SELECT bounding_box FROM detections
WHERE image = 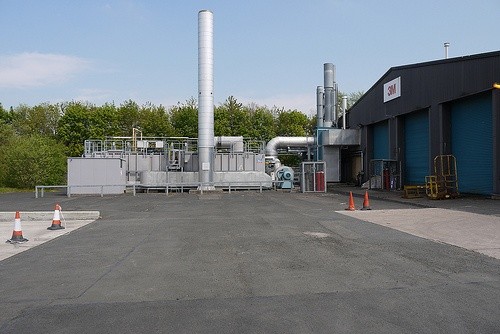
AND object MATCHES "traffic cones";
[6,210,28,244]
[360,191,372,210]
[345,192,358,211]
[47,204,65,231]
